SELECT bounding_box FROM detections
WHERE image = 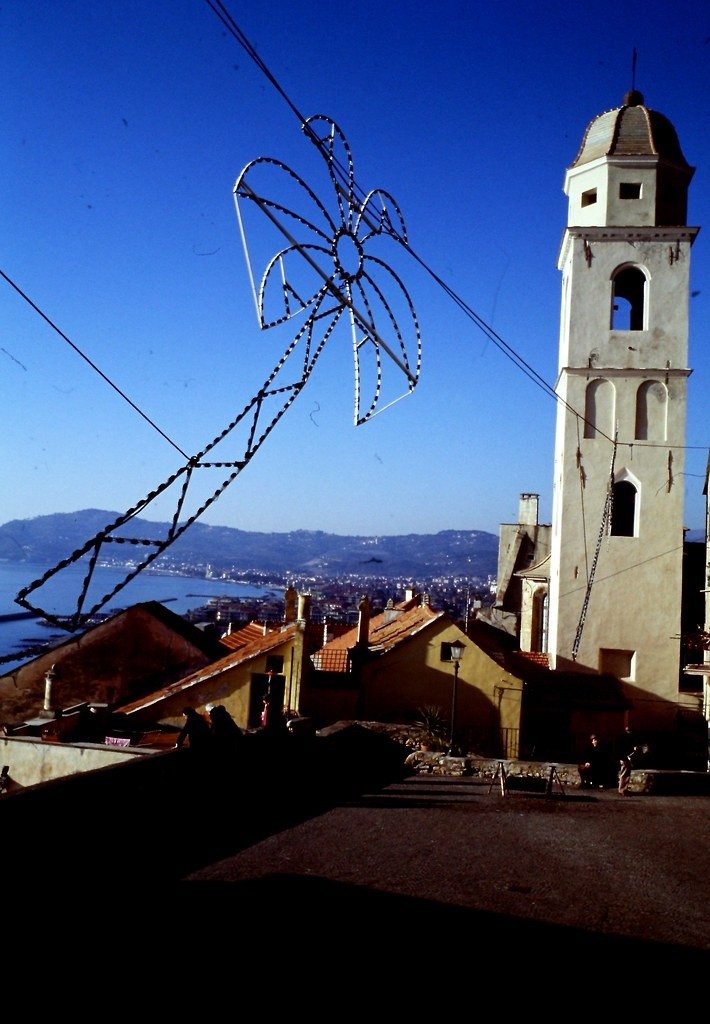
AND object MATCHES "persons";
[259,691,289,765]
[174,705,216,782]
[209,706,244,781]
[577,732,613,789]
[609,724,649,797]
